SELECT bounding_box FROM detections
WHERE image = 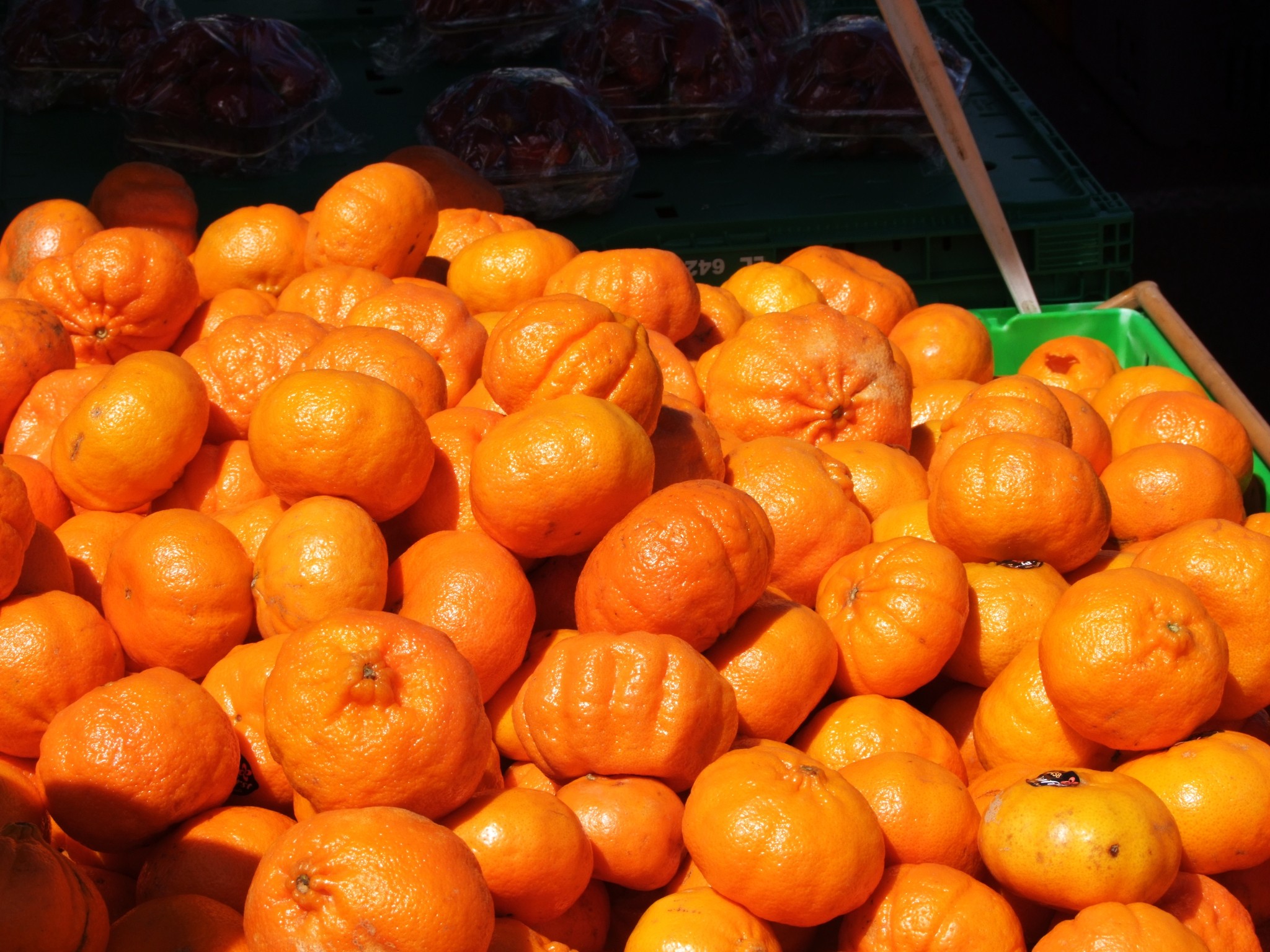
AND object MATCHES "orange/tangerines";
[0,147,1270,952]
